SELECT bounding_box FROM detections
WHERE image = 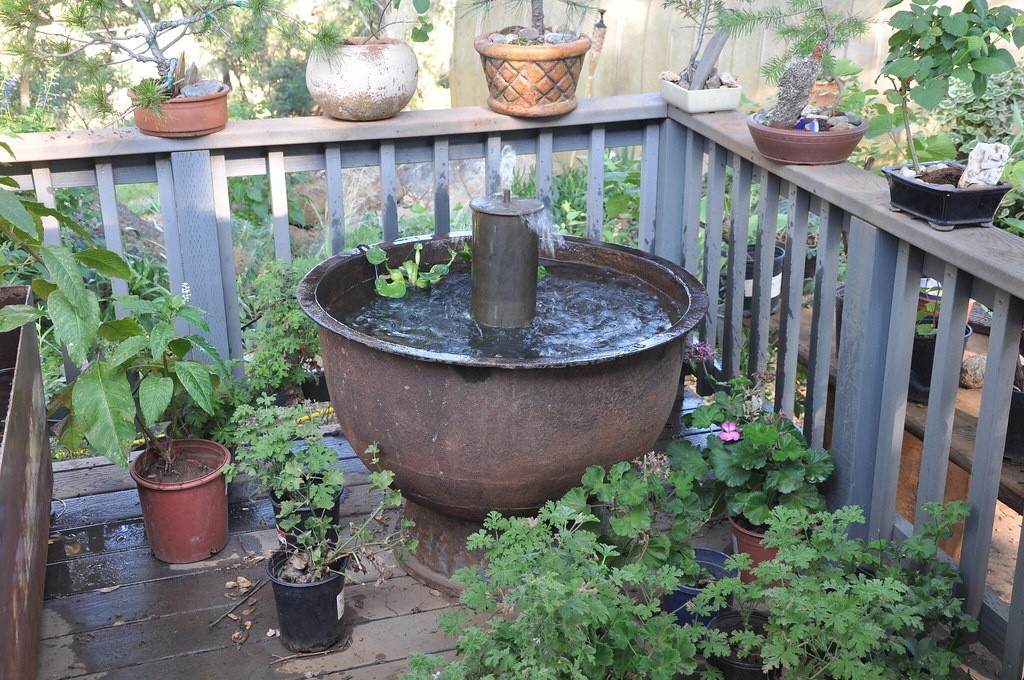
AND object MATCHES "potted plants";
[3,186,232,563]
[457,426,977,680]
[656,1,751,115]
[883,0,1024,233]
[298,229,717,519]
[124,77,233,141]
[228,404,360,649]
[307,30,421,123]
[744,3,873,169]
[908,285,975,420]
[743,238,790,321]
[477,23,594,122]
